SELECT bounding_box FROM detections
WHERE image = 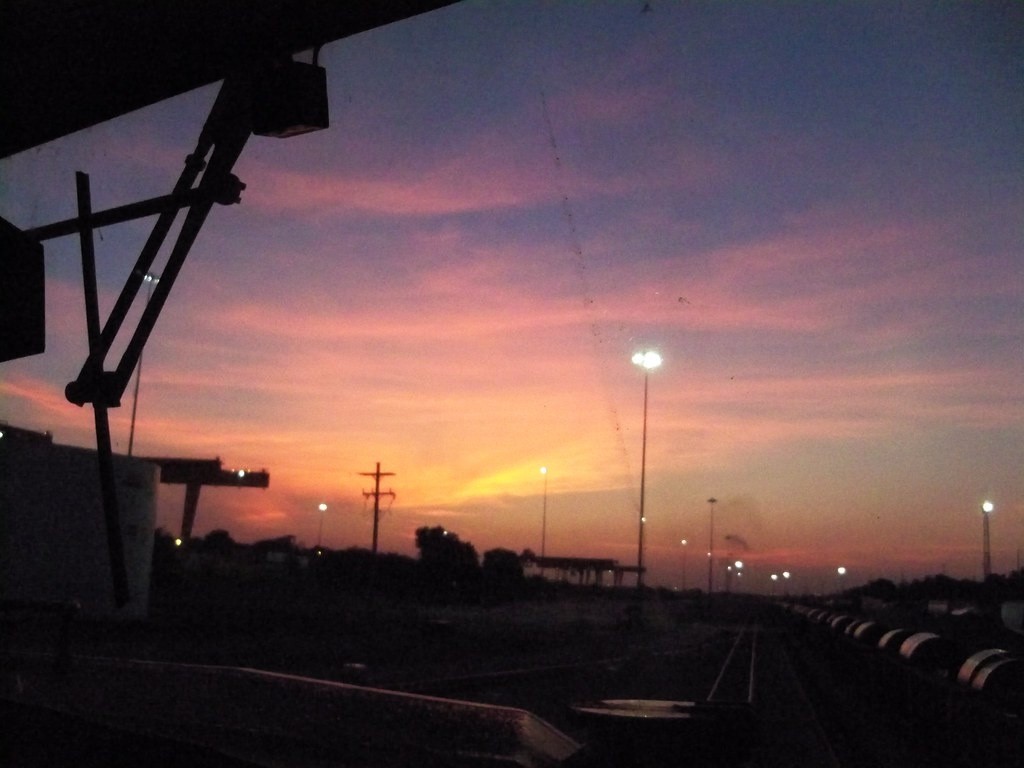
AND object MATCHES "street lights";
[632,350,663,591]
[318,503,327,547]
[681,539,688,591]
[541,467,548,575]
[708,496,717,596]
